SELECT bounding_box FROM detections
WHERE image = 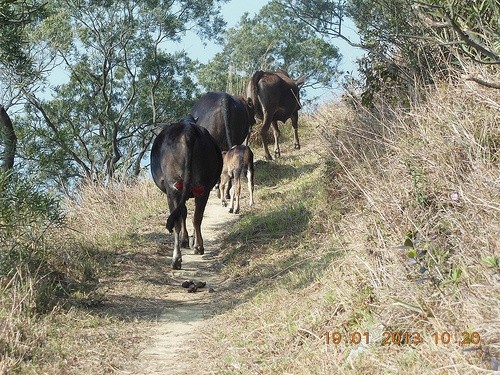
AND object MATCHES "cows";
[150,114,224,270]
[246,70,306,162]
[194,91,257,198]
[217,144,254,213]
